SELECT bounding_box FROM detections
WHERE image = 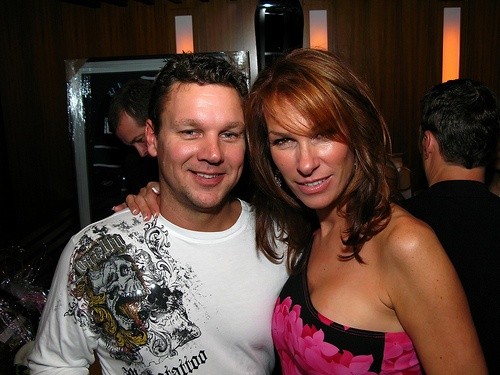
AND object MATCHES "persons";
[27,51,302,375]
[112,48,489,375]
[108,73,159,159]
[391,78,500,375]
